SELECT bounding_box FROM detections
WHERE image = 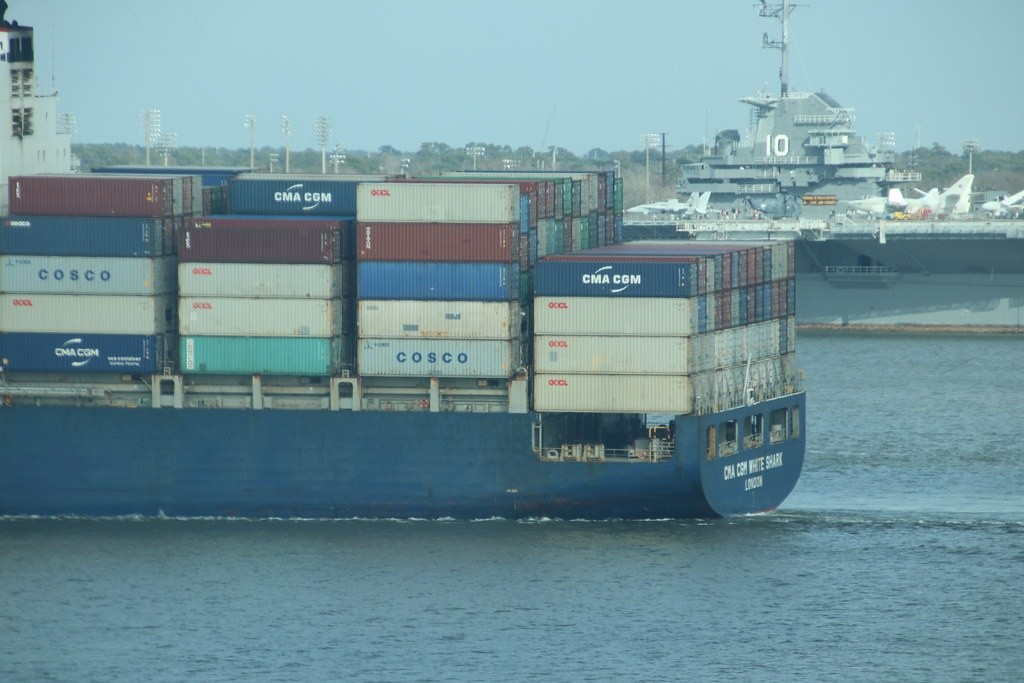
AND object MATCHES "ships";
[623,0,1023,275]
[0,163,805,521]
